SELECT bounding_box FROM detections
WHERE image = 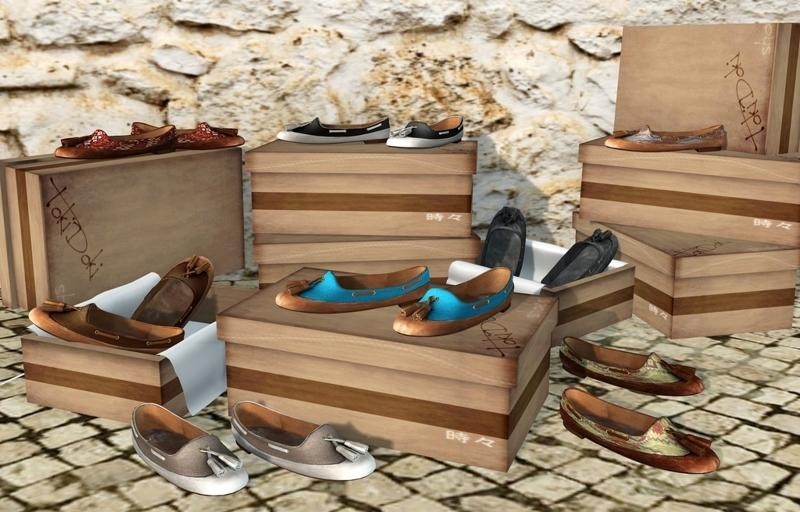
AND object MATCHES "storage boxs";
[1,22,800,475]
